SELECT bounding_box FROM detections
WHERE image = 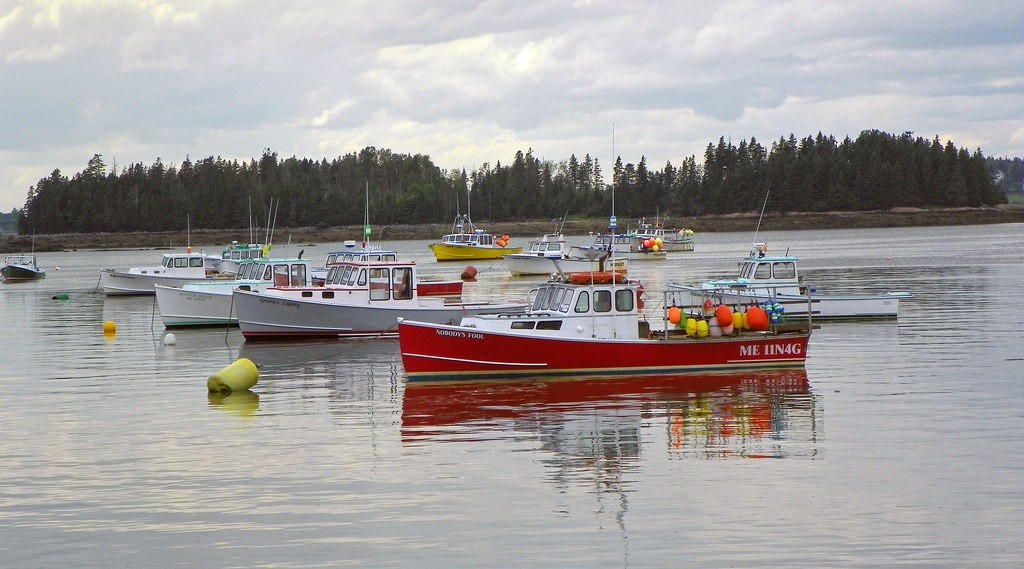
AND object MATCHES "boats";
[1,227,46,283]
[91,197,280,299]
[152,204,396,330]
[502,208,628,279]
[668,192,916,322]
[428,190,523,262]
[572,230,668,261]
[394,212,820,386]
[628,206,695,253]
[232,182,466,342]
